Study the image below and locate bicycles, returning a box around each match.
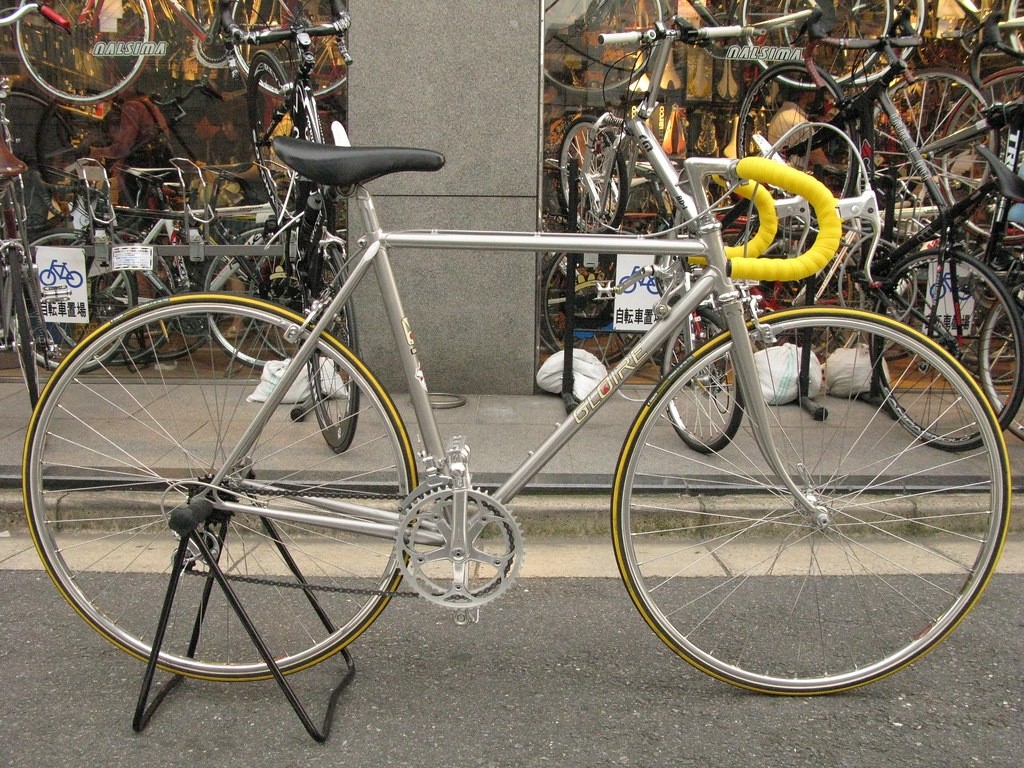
[23,136,1014,745]
[1,1,1024,457]
